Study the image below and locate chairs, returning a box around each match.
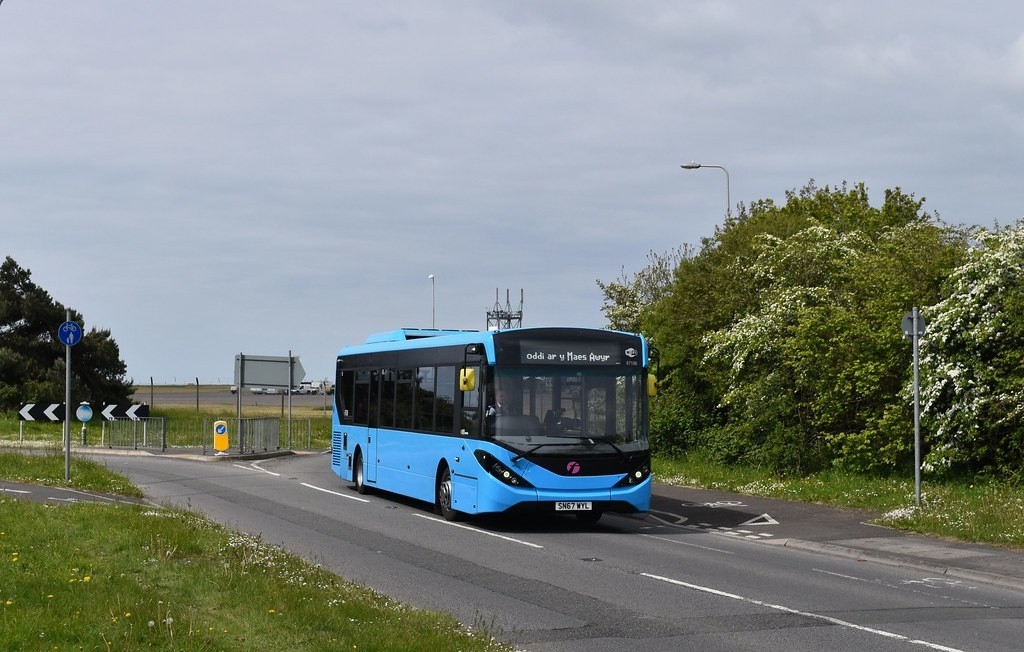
[495,415,545,436]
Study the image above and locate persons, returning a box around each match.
[493,388,521,416]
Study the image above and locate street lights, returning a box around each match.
[680,162,730,220]
[428,274,435,328]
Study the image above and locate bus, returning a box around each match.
[331,326,653,520]
